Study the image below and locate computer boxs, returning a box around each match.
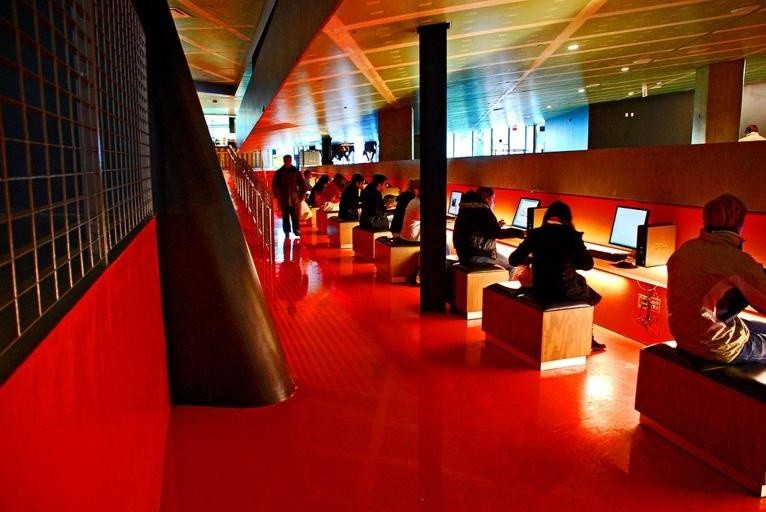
[635,222,677,267]
[527,206,548,235]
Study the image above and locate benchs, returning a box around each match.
[635,339,766,498]
[445,255,593,372]
[308,208,420,284]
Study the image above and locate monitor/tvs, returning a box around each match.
[448,189,463,218]
[608,205,650,249]
[511,197,541,231]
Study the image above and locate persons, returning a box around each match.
[391,179,416,240]
[273,239,310,316]
[307,174,329,205]
[270,155,305,239]
[302,169,314,191]
[666,192,766,368]
[508,200,607,352]
[337,173,364,221]
[358,174,394,230]
[319,173,348,212]
[451,187,528,281]
[399,180,421,244]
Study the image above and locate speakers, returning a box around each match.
[229,117,235,134]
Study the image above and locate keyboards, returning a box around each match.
[587,248,628,262]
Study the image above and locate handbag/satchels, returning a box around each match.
[369,211,388,228]
[509,262,534,288]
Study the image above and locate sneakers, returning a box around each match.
[592,339,606,349]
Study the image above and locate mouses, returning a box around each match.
[617,259,632,267]
